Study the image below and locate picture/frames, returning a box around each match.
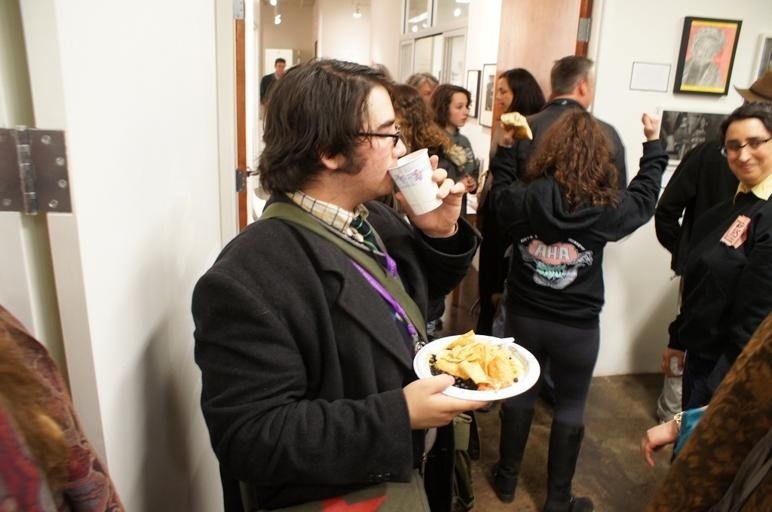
[656,106,731,167]
[675,15,741,97]
[750,33,771,86]
[464,64,496,127]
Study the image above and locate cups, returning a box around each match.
[388,148,443,216]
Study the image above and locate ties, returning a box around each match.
[350,215,384,258]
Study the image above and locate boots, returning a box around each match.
[489,405,536,504]
[543,418,593,512]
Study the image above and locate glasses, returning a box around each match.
[355,126,402,147]
[720,135,772,159]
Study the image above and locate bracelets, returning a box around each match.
[672,410,686,432]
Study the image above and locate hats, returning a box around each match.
[732,69,772,106]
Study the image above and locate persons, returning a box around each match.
[0,306,132,511]
[375,53,670,511]
[650,65,771,424]
[186,52,492,512]
[259,58,289,120]
[659,99,771,471]
[638,313,771,511]
[682,25,727,90]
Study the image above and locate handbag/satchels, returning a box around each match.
[275,469,432,511]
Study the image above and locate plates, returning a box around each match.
[412,335,540,402]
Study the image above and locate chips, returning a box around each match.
[435,329,520,385]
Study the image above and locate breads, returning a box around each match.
[500,109,534,142]
